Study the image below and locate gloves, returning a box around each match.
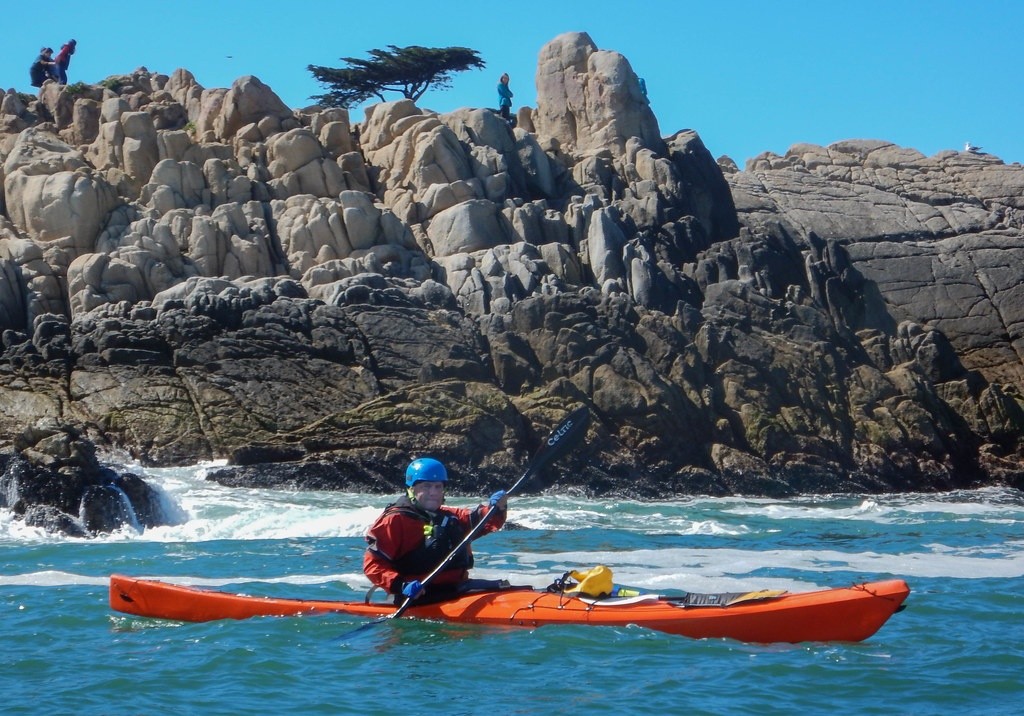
[402,580,426,600]
[488,490,508,512]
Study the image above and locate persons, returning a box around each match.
[363,457,509,607]
[29,39,77,86]
[497,73,514,121]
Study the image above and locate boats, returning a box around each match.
[109,571,909,646]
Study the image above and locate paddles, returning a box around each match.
[348,404,595,639]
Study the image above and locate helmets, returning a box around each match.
[405,458,448,486]
